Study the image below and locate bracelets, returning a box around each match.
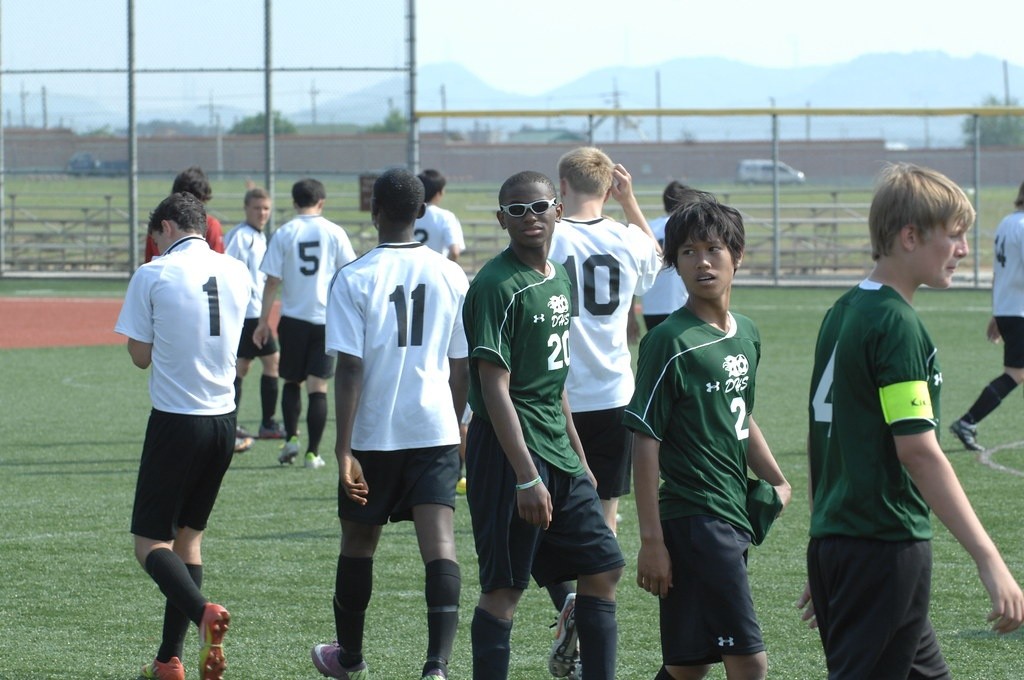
[516,476,542,491]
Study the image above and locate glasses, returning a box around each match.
[501,198,557,218]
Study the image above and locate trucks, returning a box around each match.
[65,152,128,178]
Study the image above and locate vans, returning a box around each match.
[738,159,805,188]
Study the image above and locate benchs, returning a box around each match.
[0,187,996,275]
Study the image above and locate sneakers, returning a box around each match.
[197,602,232,680]
[278,436,300,464]
[311,641,367,680]
[949,420,985,451]
[258,420,300,438]
[419,667,446,680]
[548,592,579,677]
[234,436,254,452]
[141,655,185,680]
[304,452,326,467]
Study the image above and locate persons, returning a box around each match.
[311,167,470,680]
[548,147,663,679]
[417,169,473,495]
[462,171,627,680]
[795,162,1024,680]
[641,181,689,331]
[223,188,301,452]
[112,194,253,680]
[948,182,1024,451]
[252,178,357,469]
[620,189,792,680]
[145,166,255,452]
